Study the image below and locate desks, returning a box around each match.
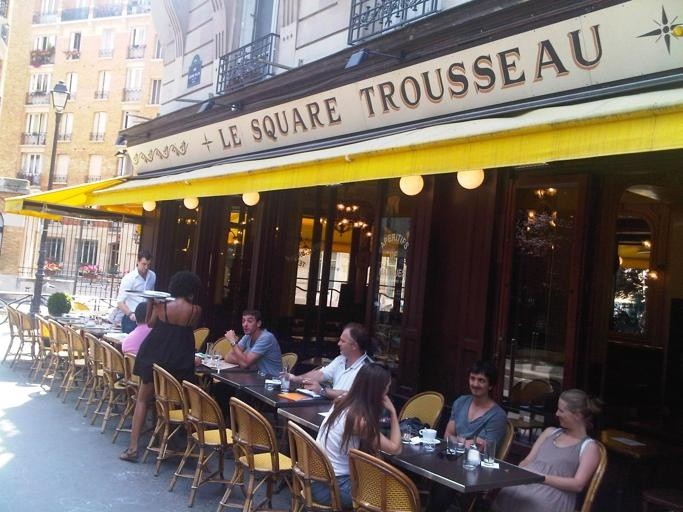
[209,370,277,391]
[278,403,330,432]
[245,385,333,407]
[398,448,548,493]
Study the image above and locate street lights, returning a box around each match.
[16,81,70,322]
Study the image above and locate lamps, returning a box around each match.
[184,198,199,210]
[143,201,156,211]
[320,185,372,237]
[457,169,485,190]
[242,192,260,206]
[399,175,424,196]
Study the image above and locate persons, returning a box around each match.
[423,360,508,512]
[491,386,606,512]
[117,250,157,334]
[224,308,284,377]
[118,269,204,462]
[278,321,376,400]
[121,301,153,356]
[309,362,405,509]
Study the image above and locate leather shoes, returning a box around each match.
[119,449,139,461]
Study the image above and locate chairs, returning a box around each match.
[142,364,211,477]
[281,353,299,372]
[397,391,444,430]
[4,306,208,442]
[169,381,238,508]
[463,418,515,507]
[287,420,344,511]
[217,398,295,511]
[575,441,608,509]
[211,336,238,359]
[348,448,422,511]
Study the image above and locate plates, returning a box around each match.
[60,310,122,331]
[141,290,171,298]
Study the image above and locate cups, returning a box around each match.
[481,438,497,465]
[461,443,481,470]
[397,423,466,457]
[193,340,227,376]
[255,363,293,395]
[467,472,481,487]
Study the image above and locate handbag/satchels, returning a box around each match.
[400,417,429,437]
